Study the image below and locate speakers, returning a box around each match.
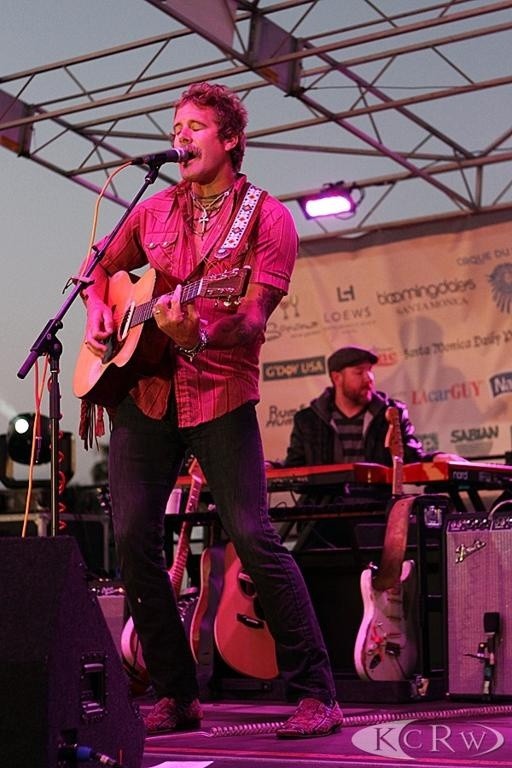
[1,536,145,767]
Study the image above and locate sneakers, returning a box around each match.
[274,696,344,739]
[143,694,205,734]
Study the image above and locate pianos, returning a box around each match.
[385,454,511,493]
[174,460,387,495]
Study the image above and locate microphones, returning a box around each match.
[132,147,196,166]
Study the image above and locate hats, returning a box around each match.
[327,346,380,374]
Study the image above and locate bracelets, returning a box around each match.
[176,335,207,357]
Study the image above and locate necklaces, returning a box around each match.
[190,185,232,235]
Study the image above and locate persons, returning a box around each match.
[266,347,425,552]
[79,81,344,739]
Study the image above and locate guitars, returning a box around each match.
[184,505,204,666]
[199,542,283,688]
[70,267,250,409]
[119,456,209,681]
[354,406,420,681]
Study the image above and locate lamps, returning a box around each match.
[300,187,355,220]
